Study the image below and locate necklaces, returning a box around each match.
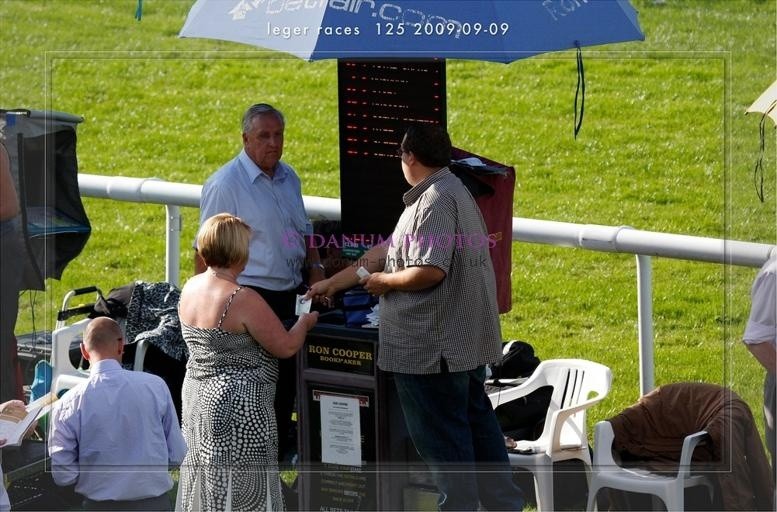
[207,269,235,279]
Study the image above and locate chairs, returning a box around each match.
[587,382,740,511]
[49,283,178,395]
[14,285,102,366]
[488,358,613,511]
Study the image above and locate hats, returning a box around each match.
[401,124,452,169]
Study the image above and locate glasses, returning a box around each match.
[397,148,405,155]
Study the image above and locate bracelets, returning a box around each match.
[307,260,326,272]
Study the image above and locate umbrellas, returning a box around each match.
[177,0,645,64]
[744,80,776,129]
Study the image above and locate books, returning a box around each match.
[0,399,44,448]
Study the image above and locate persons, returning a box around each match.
[192,102,328,470]
[0,141,28,403]
[0,438,12,512]
[46,316,189,512]
[303,120,526,511]
[174,212,320,512]
[741,255,776,475]
